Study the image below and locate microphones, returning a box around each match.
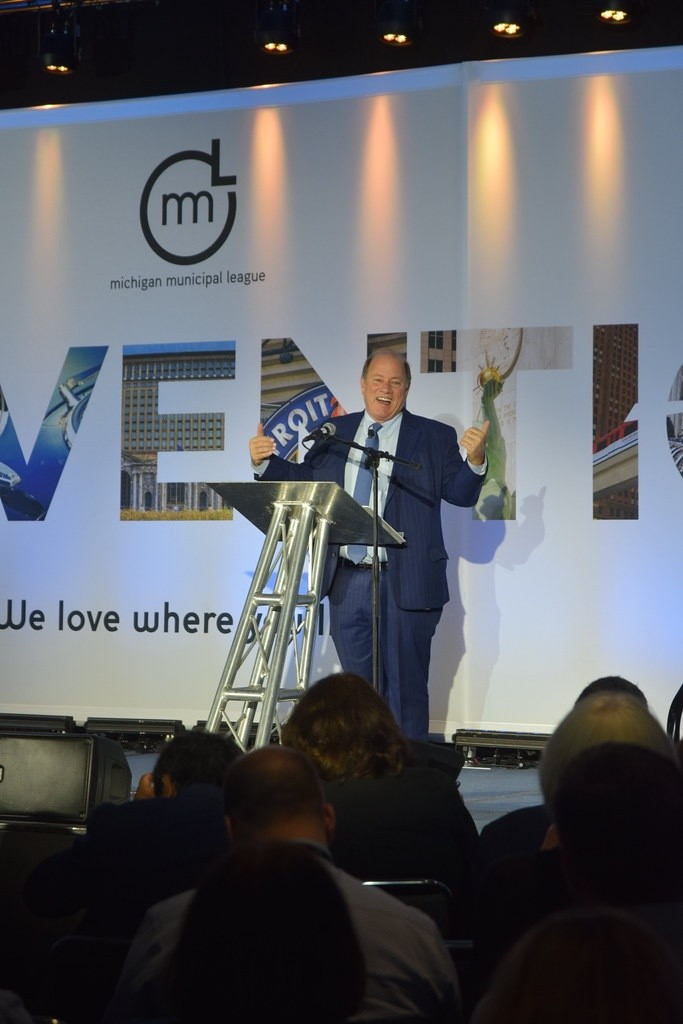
[303,423,337,443]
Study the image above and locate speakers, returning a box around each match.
[0,731,134,828]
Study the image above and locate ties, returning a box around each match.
[346,423,382,565]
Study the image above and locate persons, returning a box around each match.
[467,690,683,935]
[99,745,464,1024]
[284,674,479,893]
[22,732,245,938]
[473,907,683,1024]
[554,744,683,956]
[168,841,368,1024]
[249,350,491,740]
[469,677,683,884]
